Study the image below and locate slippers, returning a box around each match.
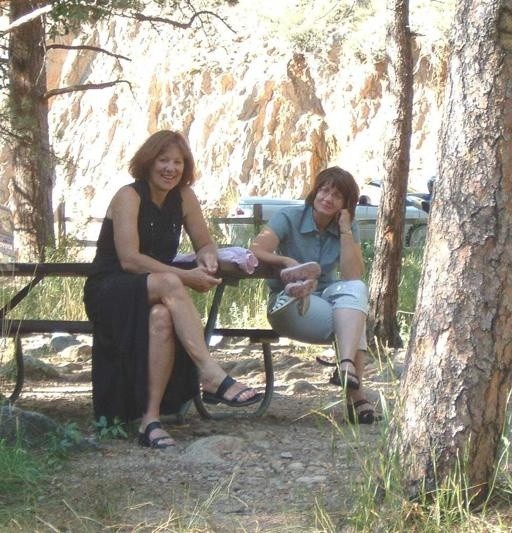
[203,376,263,407]
[139,422,176,448]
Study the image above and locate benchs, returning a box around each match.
[0,315,204,407]
[210,325,279,419]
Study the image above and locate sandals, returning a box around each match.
[268,261,321,316]
[348,399,383,422]
[330,359,360,388]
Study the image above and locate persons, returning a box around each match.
[251,166,382,424]
[83,129,265,449]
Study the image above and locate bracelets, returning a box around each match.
[340,230,352,235]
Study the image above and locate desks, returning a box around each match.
[0,254,286,421]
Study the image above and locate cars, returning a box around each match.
[178,175,435,249]
